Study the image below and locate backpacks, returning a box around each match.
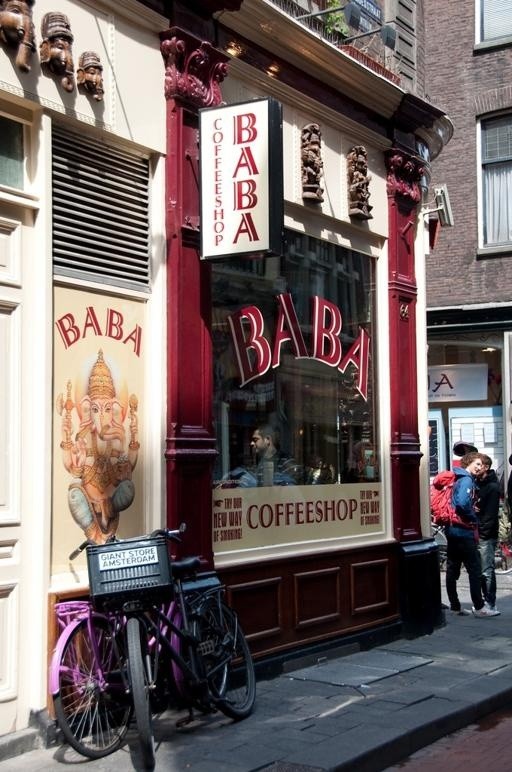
[429,470,475,529]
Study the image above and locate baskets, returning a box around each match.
[86,536,172,606]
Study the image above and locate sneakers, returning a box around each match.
[451,608,472,616]
[472,601,501,617]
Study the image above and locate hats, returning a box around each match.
[454,441,478,456]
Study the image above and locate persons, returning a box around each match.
[470,454,501,611]
[445,452,502,618]
[239,425,302,485]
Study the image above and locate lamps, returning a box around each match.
[293,1,398,53]
